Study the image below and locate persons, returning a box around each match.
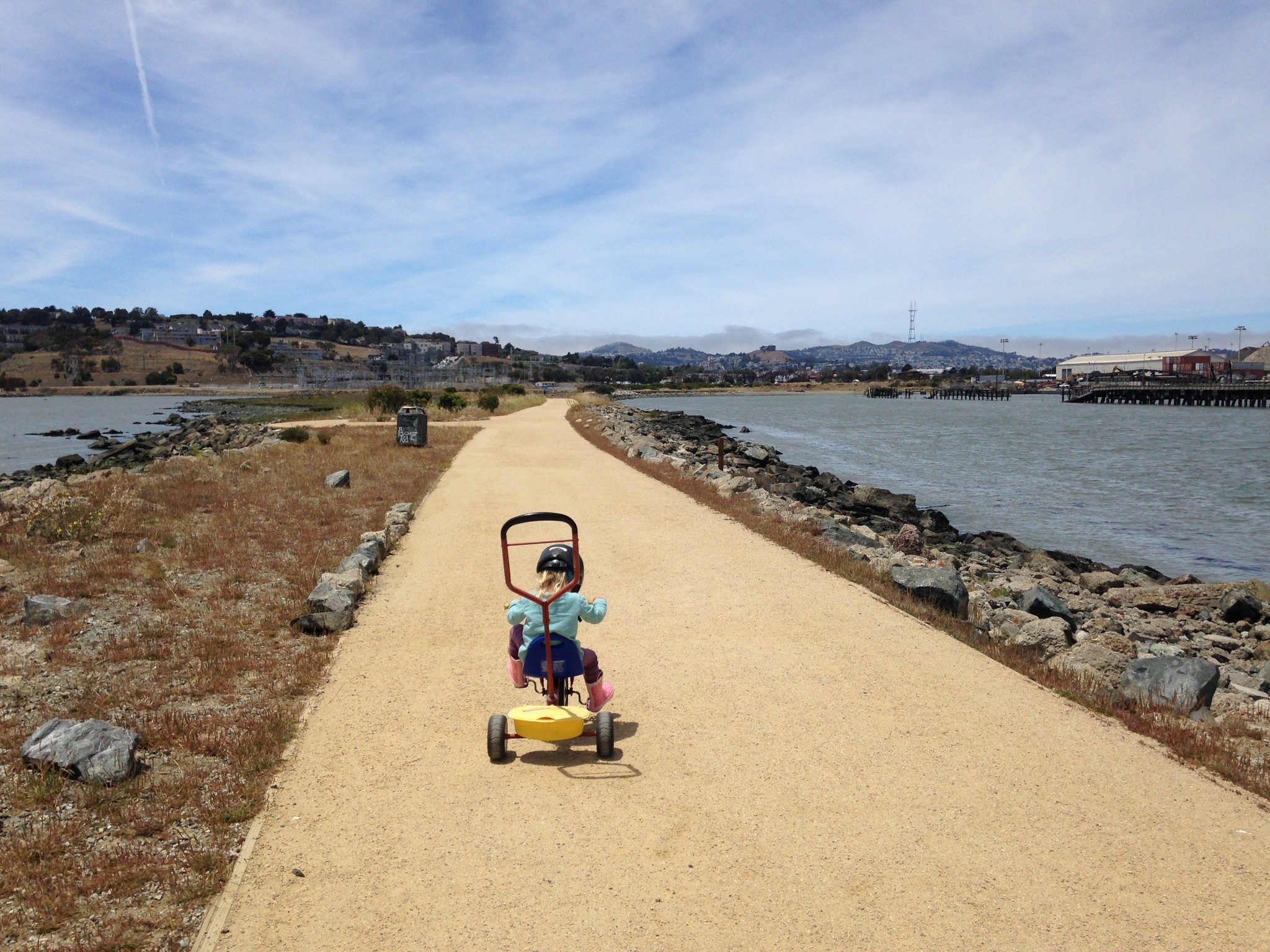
[506,544,614,713]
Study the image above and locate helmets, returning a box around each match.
[536,544,584,593]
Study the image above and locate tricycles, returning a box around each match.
[486,511,616,760]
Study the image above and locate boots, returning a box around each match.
[507,652,527,688]
[585,669,614,713]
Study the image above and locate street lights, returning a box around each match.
[1188,335,1198,350]
[1039,342,1042,377]
[1234,325,1247,362]
[1000,338,1009,374]
